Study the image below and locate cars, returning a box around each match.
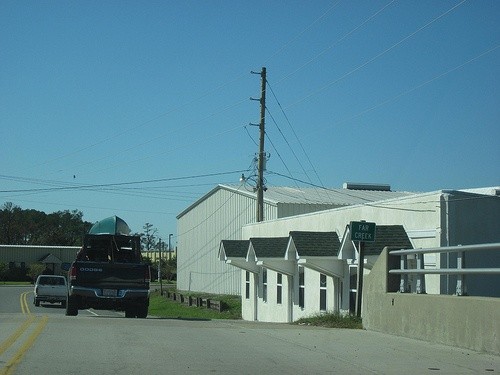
[33,275,67,307]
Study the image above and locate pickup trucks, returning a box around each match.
[65,234,152,320]
